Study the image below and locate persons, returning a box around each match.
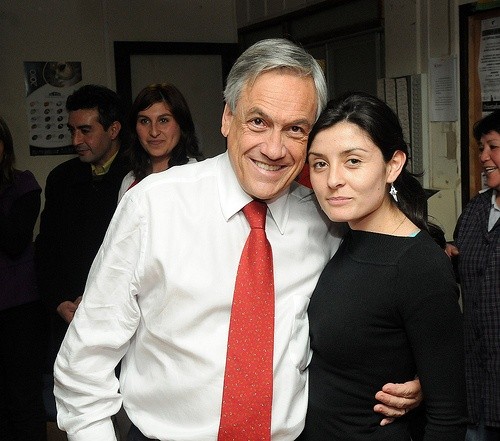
[116,82,204,206]
[445,108,500,441]
[53,38,421,441]
[306,91,469,441]
[0,116,47,441]
[30,84,136,396]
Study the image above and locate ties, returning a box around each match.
[218,201,275,441]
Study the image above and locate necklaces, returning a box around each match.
[391,216,407,233]
